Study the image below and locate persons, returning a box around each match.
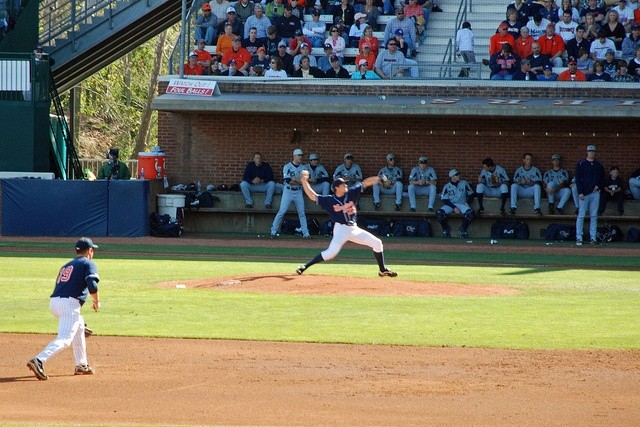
[333,153,363,187]
[268,148,312,240]
[475,158,511,217]
[178,0,444,78]
[575,144,601,247]
[296,170,398,277]
[543,153,572,215]
[96,148,131,180]
[482,0,640,82]
[436,168,475,239]
[407,157,438,212]
[629,175,640,200]
[597,163,624,216]
[569,175,579,216]
[510,153,542,217]
[372,153,405,211]
[240,151,276,209]
[304,153,330,196]
[27,236,100,381]
[455,21,476,77]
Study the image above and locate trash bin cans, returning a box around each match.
[157,194,185,237]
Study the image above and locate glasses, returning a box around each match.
[287,8,292,11]
[331,30,338,32]
[503,29,507,31]
[419,160,428,164]
[397,13,403,15]
[363,64,368,66]
[595,65,602,66]
[390,158,395,161]
[390,44,396,45]
[314,13,320,16]
[270,62,276,64]
[205,10,211,12]
[527,63,531,66]
[225,21,231,25]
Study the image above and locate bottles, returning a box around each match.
[84,168,89,180]
[229,64,234,75]
[197,179,202,191]
[140,168,145,178]
[525,71,530,80]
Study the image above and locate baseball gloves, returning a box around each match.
[487,173,502,187]
[79,322,95,342]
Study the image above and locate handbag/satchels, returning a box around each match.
[391,219,431,237]
[321,219,335,236]
[283,218,320,235]
[588,224,623,241]
[490,220,530,240]
[357,217,391,236]
[184,191,214,207]
[545,223,584,242]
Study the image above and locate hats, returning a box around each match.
[586,145,596,151]
[227,7,236,13]
[354,12,366,20]
[362,42,371,48]
[499,23,508,28]
[189,51,198,57]
[418,157,428,161]
[448,169,460,177]
[547,24,555,27]
[333,178,349,186]
[110,149,118,154]
[386,153,394,158]
[607,49,615,54]
[278,42,286,47]
[395,29,403,35]
[301,42,308,48]
[631,24,640,29]
[344,154,353,159]
[75,236,99,251]
[293,149,303,154]
[502,43,512,49]
[258,47,265,51]
[335,16,343,23]
[324,43,332,49]
[228,59,236,64]
[232,36,241,41]
[310,153,318,159]
[552,154,560,159]
[331,55,338,62]
[312,9,320,14]
[543,65,552,69]
[199,39,206,44]
[284,3,292,8]
[203,3,211,10]
[569,60,577,64]
[359,59,366,65]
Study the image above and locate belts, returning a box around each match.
[287,187,301,191]
[488,186,500,188]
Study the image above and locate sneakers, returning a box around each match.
[459,227,468,239]
[245,202,254,208]
[534,208,542,216]
[74,364,94,375]
[590,240,599,246]
[395,204,402,212]
[576,240,582,246]
[510,208,517,215]
[373,202,382,211]
[296,265,305,275]
[270,230,277,238]
[442,225,451,238]
[378,268,398,277]
[302,232,311,238]
[266,203,272,209]
[27,357,48,381]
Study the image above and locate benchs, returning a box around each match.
[185,186,639,238]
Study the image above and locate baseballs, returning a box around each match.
[302,170,309,176]
